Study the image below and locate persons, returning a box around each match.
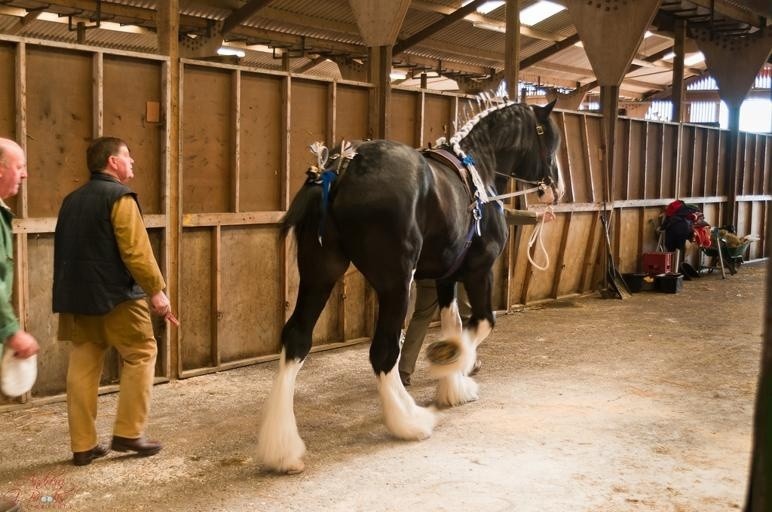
[50,134,172,467]
[0,136,43,358]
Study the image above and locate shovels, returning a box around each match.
[600,217,633,299]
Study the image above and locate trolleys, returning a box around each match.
[703,242,750,274]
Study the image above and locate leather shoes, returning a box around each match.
[73,444,111,465]
[111,436,163,453]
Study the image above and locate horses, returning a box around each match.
[255,96,568,476]
